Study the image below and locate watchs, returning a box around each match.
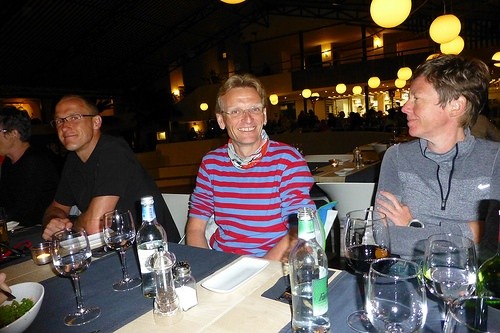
[408,219,424,229]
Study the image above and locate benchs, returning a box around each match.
[160,183,375,259]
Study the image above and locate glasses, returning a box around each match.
[221,106,263,118]
[50,113,96,130]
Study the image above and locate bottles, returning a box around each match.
[145,246,180,317]
[474,210,500,333]
[173,261,198,312]
[289,207,331,333]
[135,196,169,298]
[353,146,362,170]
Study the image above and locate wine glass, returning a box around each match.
[102,209,142,292]
[50,227,101,326]
[422,233,476,323]
[343,210,391,333]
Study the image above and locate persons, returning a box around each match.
[373,54,500,263]
[187,106,407,141]
[186,74,317,264]
[42,94,181,244]
[470,113,500,142]
[0,105,64,227]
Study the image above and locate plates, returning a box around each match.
[201,256,270,293]
[334,167,355,176]
[59,231,103,248]
[0,221,19,233]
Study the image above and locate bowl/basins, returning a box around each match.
[0,282,44,333]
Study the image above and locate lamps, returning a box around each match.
[370,0,412,28]
[429,0,465,55]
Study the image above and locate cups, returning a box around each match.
[0,219,9,245]
[366,258,428,333]
[30,241,51,265]
[292,208,327,299]
[442,296,500,333]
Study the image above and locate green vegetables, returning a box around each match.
[0,297,33,331]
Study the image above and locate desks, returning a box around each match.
[312,158,380,187]
[0,227,445,333]
